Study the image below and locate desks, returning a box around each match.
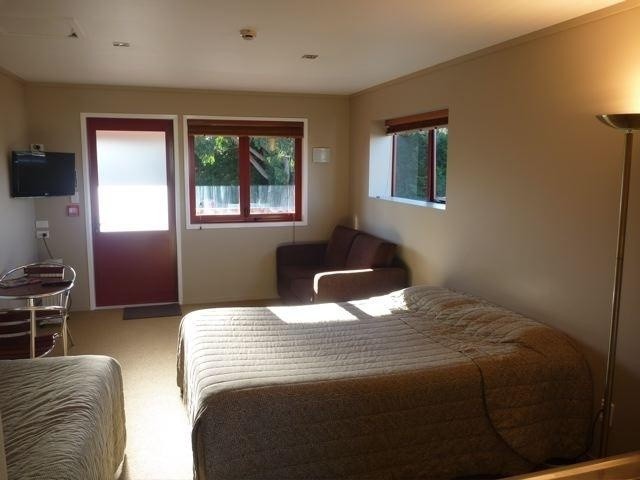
[0,276,74,360]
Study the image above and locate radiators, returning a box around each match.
[39,256,64,317]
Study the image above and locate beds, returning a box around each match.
[172,283,603,479]
[1,355,129,479]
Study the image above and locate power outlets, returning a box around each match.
[37,230,50,239]
[597,397,616,427]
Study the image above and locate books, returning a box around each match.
[0,276,72,290]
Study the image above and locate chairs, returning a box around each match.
[0,260,77,347]
[0,304,67,360]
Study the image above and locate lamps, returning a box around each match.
[585,107,640,462]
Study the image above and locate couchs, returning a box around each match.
[274,225,408,306]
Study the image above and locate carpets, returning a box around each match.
[121,301,183,320]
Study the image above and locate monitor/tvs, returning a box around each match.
[8,150,75,198]
